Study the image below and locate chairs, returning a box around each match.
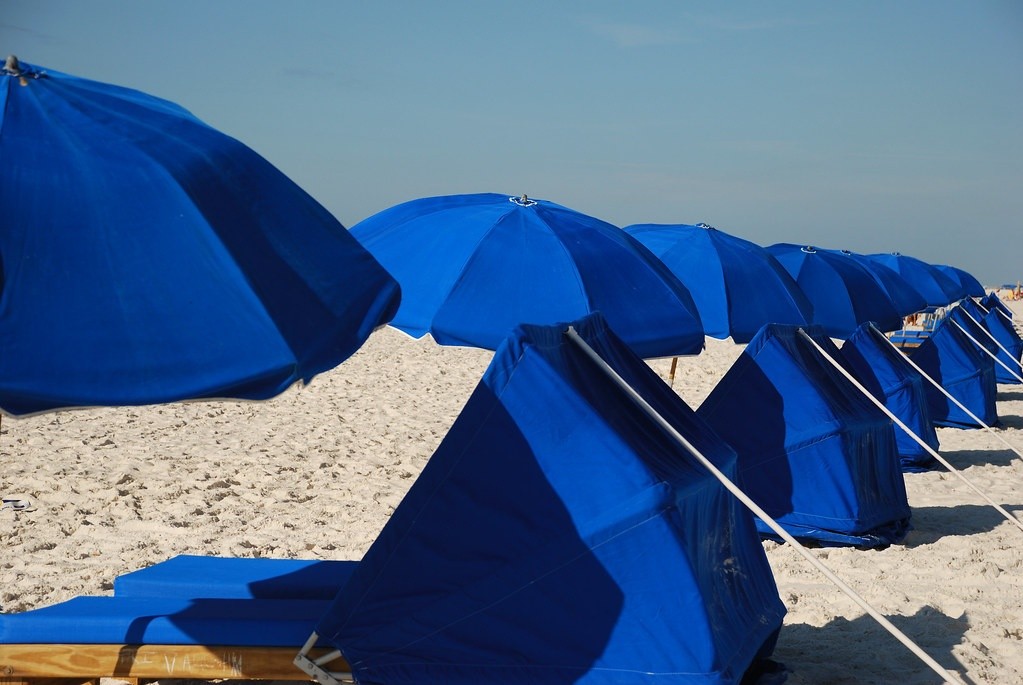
[0,552,357,684]
[888,328,930,352]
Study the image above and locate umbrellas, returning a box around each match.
[762,243,986,341]
[617,222,814,345]
[0,56,402,421]
[345,193,706,360]
